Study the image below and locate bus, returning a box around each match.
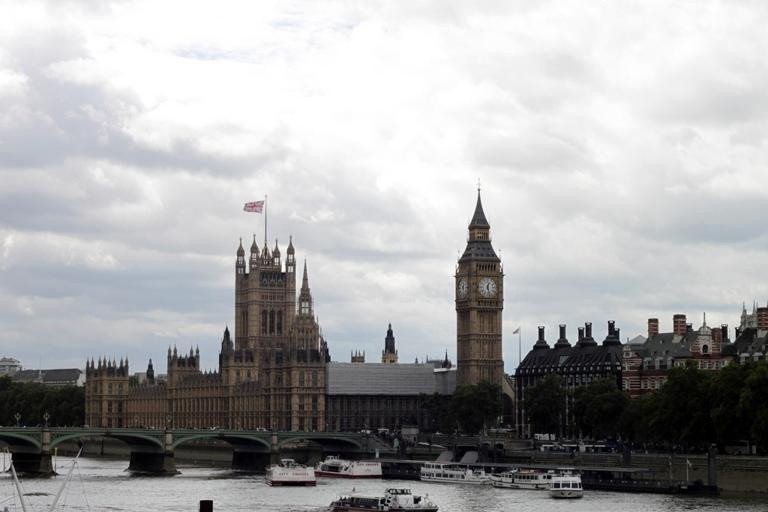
[711,438,751,456]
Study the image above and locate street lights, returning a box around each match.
[14,412,22,427]
[43,410,50,427]
[432,417,436,433]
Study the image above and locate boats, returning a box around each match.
[315,455,383,479]
[486,467,551,490]
[265,458,317,488]
[363,457,424,480]
[672,459,722,495]
[575,464,672,491]
[546,470,585,498]
[327,485,440,512]
[420,461,491,485]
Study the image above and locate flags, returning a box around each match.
[512,328,520,335]
[243,199,265,214]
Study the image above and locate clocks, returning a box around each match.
[458,278,497,298]
